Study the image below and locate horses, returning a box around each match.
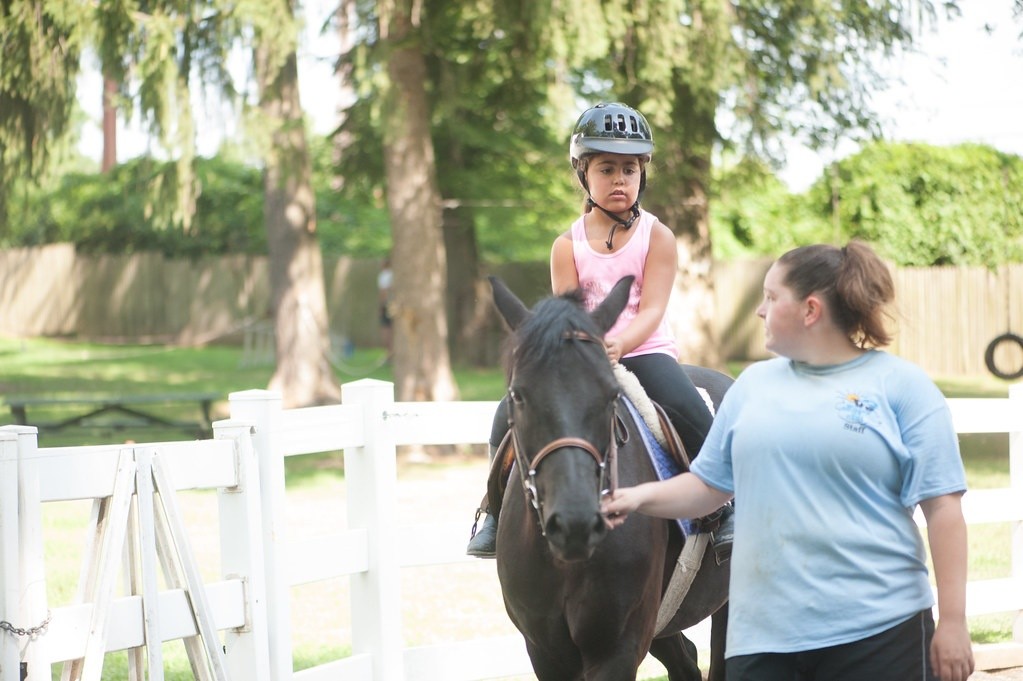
[486,275,735,681]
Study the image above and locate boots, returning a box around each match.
[467,444,500,557]
[714,505,734,554]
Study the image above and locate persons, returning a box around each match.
[598,241,974,681]
[466,102,735,566]
[375,256,396,364]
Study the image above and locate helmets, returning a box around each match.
[569,101,654,172]
